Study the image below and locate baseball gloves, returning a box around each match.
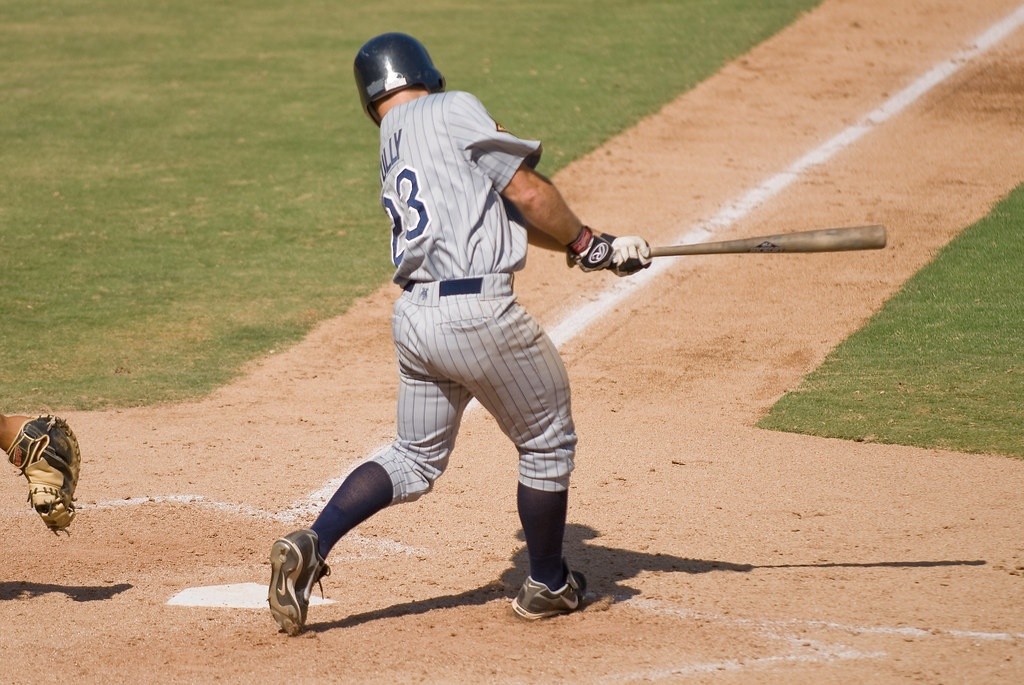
[6,415,80,537]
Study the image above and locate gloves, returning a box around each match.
[565,226,615,271]
[600,233,651,277]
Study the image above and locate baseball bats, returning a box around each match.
[565,223,887,265]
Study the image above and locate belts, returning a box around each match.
[403,278,482,296]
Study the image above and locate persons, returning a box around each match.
[266,32,652,638]
[0,414,46,470]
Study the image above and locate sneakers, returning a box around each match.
[269,529,331,636]
[512,557,587,620]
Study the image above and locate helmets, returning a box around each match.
[354,32,445,127]
